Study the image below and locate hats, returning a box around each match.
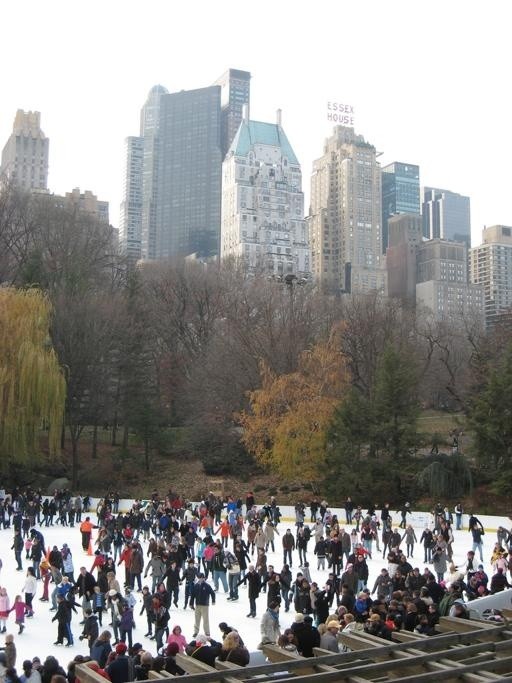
[295,613,304,621]
[359,591,366,597]
[367,613,380,622]
[116,643,126,654]
[328,621,342,629]
[168,642,178,652]
[269,602,278,608]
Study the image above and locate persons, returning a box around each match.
[1,482,512,682]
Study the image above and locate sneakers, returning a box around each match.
[65,643,72,646]
[39,597,49,601]
[54,641,63,644]
[174,602,195,610]
[150,636,155,640]
[1,627,6,633]
[19,625,24,634]
[193,633,197,637]
[227,597,237,600]
